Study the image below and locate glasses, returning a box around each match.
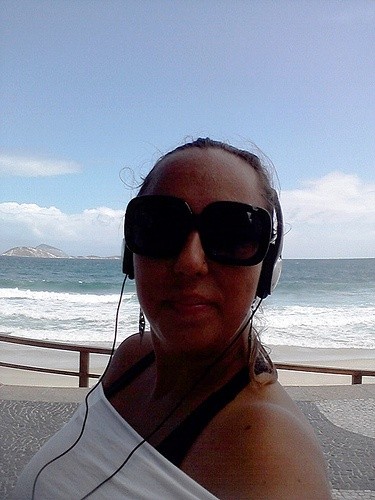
[124,194,273,267]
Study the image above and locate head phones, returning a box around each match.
[122,186,284,298]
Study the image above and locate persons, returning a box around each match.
[14,138,332,500]
